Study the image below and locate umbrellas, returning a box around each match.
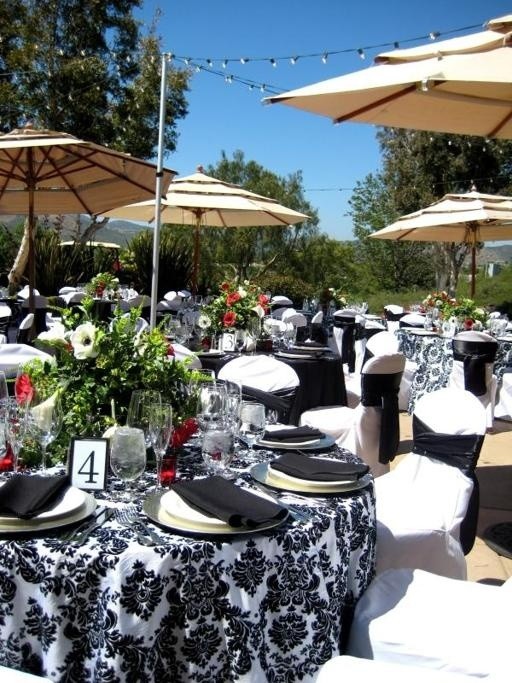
[365,183,512,298]
[258,12,512,140]
[0,121,180,336]
[93,164,313,299]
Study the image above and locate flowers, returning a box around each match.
[209,279,272,331]
[91,270,119,291]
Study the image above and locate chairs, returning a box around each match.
[349,565,512,683]
[315,656,512,683]
[1,284,512,426]
[296,351,408,479]
[369,387,490,580]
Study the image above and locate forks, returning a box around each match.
[114,506,163,546]
[44,507,110,545]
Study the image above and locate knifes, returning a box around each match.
[244,480,308,524]
[72,507,117,549]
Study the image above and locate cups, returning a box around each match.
[189,369,278,473]
[164,319,188,344]
[125,389,162,449]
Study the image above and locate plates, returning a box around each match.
[249,457,371,494]
[253,432,335,451]
[276,351,312,360]
[140,482,290,537]
[1,485,98,532]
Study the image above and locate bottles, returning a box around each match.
[209,333,222,350]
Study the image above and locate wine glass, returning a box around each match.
[185,311,204,345]
[109,403,173,502]
[4,385,64,476]
[283,330,297,350]
